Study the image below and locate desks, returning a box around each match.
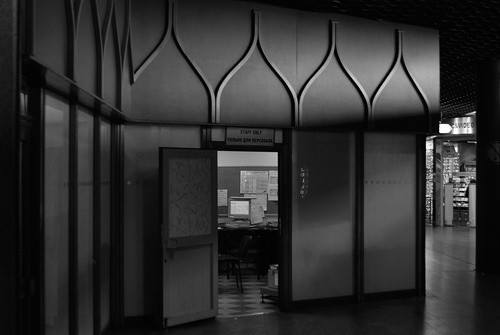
[217,223,280,280]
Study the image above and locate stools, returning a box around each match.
[218,253,243,292]
